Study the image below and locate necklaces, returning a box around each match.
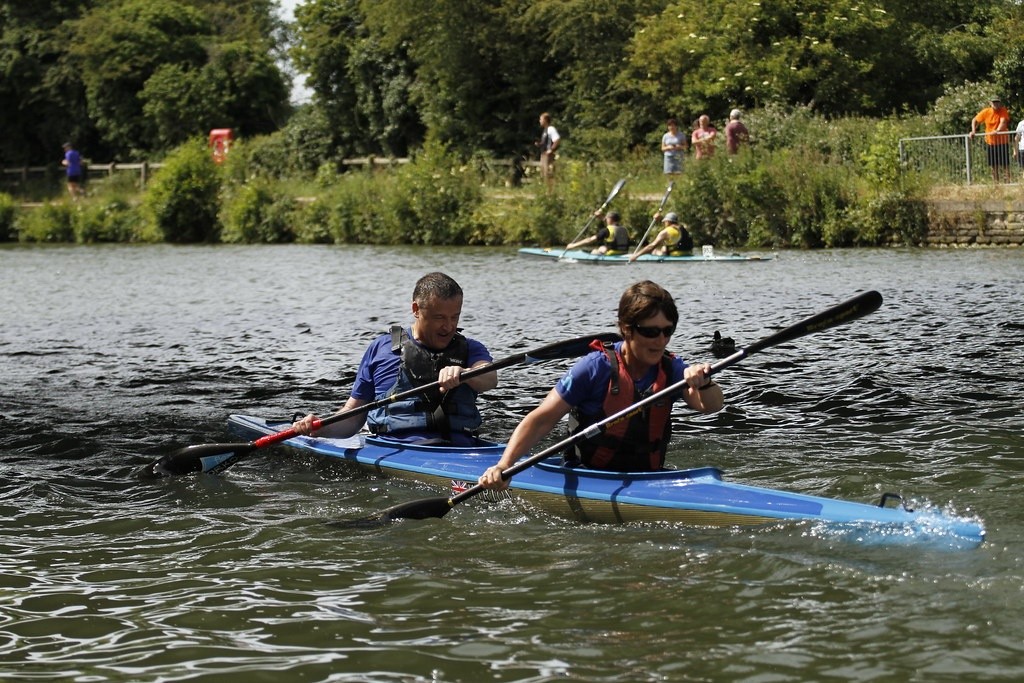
[635,383,650,418]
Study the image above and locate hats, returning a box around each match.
[989,95,1001,102]
[661,212,678,222]
[603,211,621,221]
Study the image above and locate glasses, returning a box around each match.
[635,325,676,337]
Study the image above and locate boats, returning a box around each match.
[222,413,986,554]
[516,247,781,264]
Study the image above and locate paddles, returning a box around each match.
[373,289,883,518]
[155,331,620,475]
[551,176,628,264]
[627,180,675,266]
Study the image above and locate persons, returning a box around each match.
[535,110,562,197]
[630,211,692,260]
[1013,116,1024,171]
[969,97,1011,184]
[62,139,85,204]
[726,109,749,154]
[660,118,688,191]
[566,209,629,255]
[477,282,723,491]
[691,114,717,161]
[292,272,498,439]
